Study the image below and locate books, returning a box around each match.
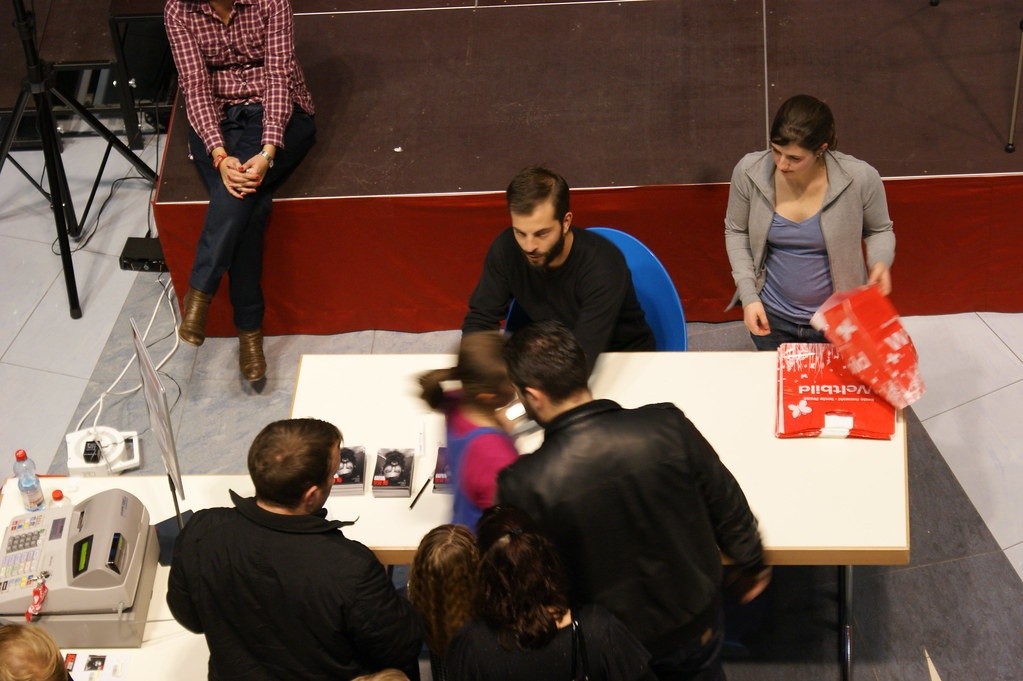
[432,446,455,495]
[330,445,367,496]
[371,447,414,498]
[496,394,537,438]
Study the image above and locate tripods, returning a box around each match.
[0,0,158,319]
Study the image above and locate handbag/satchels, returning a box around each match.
[811,284,925,410]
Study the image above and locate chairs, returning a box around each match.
[504,228,688,352]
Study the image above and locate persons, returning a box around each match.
[163,0,318,384]
[461,165,658,386]
[497,313,767,681]
[348,518,659,681]
[335,448,356,481]
[381,450,405,483]
[168,419,426,681]
[422,330,525,531]
[725,94,896,351]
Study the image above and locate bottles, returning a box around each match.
[14,450,46,513]
[49,490,73,508]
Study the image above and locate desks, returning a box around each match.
[1,475,256,680]
[287,352,910,680]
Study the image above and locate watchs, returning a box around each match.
[259,151,274,168]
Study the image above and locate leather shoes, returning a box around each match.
[177,287,212,346]
[238,329,267,382]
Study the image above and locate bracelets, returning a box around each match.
[213,153,227,168]
